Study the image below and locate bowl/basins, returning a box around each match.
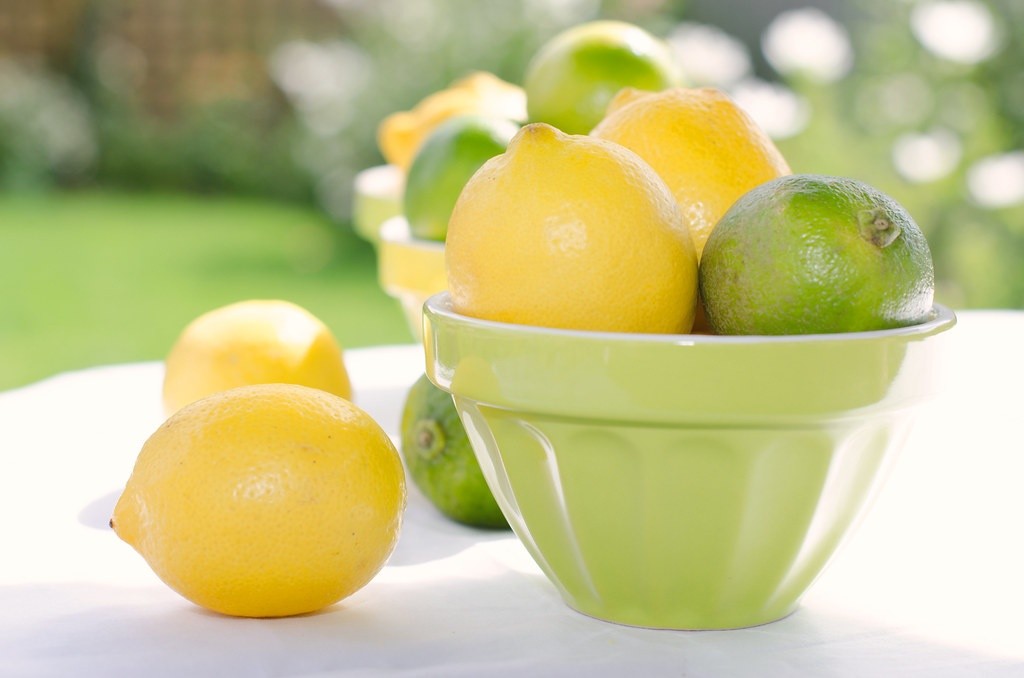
[421,289,956,631]
[375,214,445,339]
